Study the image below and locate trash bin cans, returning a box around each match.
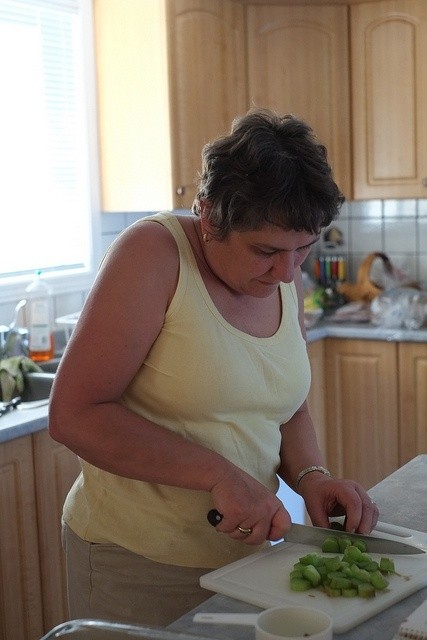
[293,464,331,493]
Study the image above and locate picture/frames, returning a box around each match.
[127,449,425,640]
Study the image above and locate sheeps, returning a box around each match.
[0,325,29,358]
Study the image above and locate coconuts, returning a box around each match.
[289,523,396,600]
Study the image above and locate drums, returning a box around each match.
[207,509,426,554]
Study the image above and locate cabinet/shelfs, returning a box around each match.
[25,266,55,361]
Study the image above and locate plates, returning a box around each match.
[248,1,353,211]
[305,329,324,473]
[347,0,426,203]
[0,417,47,639]
[398,332,427,469]
[325,329,399,490]
[33,416,91,633]
[95,0,244,212]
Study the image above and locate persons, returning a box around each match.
[48,106,379,629]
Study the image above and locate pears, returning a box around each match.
[24,269,55,362]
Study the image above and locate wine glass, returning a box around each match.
[236,526,251,537]
[370,500,376,504]
